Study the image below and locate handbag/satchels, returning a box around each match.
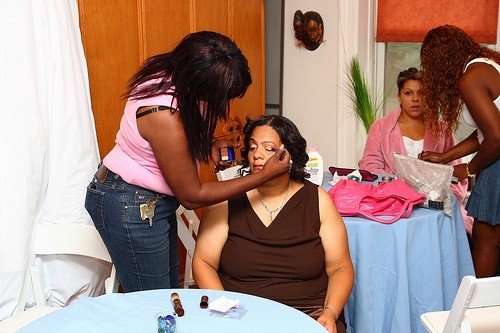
[325,178,426,225]
[393,151,454,218]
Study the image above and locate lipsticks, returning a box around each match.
[170,292,185,317]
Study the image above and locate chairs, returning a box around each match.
[0,217,116,333]
[420,274,500,333]
[175,203,200,288]
[216,164,250,181]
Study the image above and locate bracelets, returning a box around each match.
[466,164,476,178]
[323,306,339,320]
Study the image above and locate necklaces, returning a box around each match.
[257,179,291,222]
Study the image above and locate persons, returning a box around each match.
[192,114,355,333]
[416,23,500,278]
[84,29,295,295]
[356,67,474,240]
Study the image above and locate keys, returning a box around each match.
[141,204,155,226]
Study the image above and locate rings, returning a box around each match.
[290,160,293,164]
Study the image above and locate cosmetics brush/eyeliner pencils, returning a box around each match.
[270,146,286,153]
[419,150,424,160]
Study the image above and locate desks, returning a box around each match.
[16,287,330,333]
[319,171,477,332]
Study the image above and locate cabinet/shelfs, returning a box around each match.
[78,0,266,292]
